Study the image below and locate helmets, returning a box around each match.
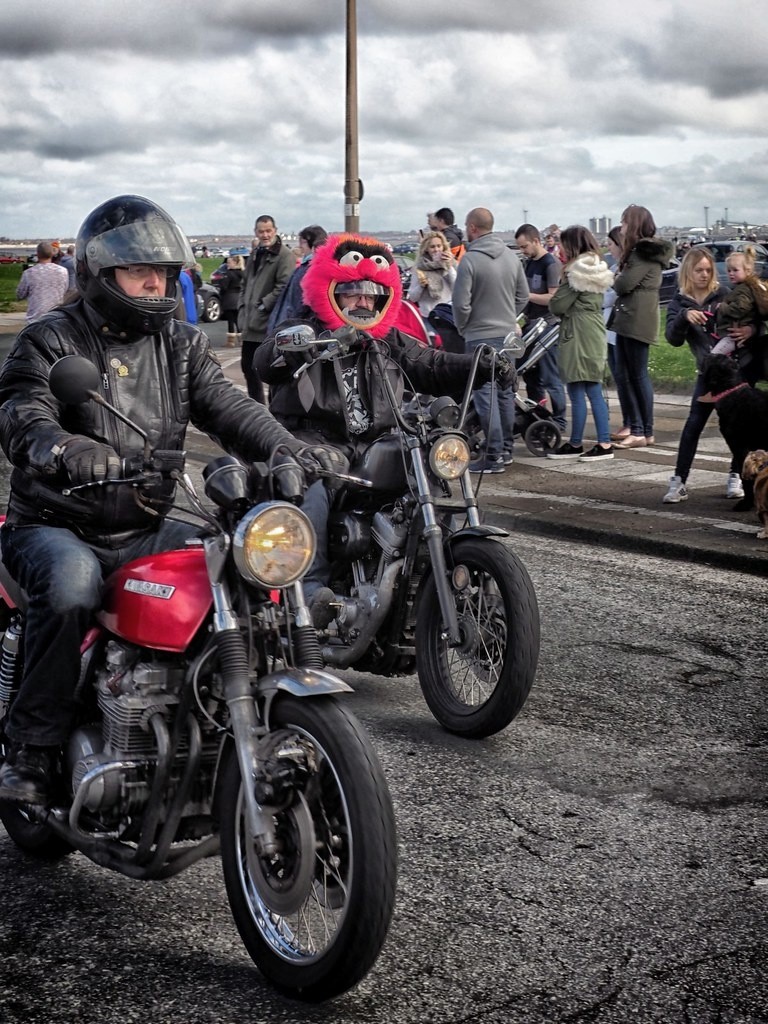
[75,196,196,333]
[334,281,389,319]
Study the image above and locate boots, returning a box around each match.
[237,333,243,347]
[222,332,236,348]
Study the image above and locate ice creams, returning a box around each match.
[415,269,425,282]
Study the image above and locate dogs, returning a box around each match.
[742,449,768,540]
[698,352,768,515]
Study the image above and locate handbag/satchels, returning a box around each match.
[430,303,454,325]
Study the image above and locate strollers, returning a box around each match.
[427,299,564,457]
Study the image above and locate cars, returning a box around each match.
[0,251,26,264]
[392,241,420,292]
[192,241,249,324]
[602,233,768,305]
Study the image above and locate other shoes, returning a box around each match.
[698,392,715,403]
[614,437,646,448]
[646,436,655,444]
[609,433,629,439]
[307,587,336,644]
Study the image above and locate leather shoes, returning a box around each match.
[0,740,59,805]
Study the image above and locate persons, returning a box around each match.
[237,215,296,404]
[662,245,768,502]
[740,234,756,242]
[0,196,351,806]
[547,224,614,461]
[407,207,677,448]
[16,241,79,329]
[252,231,519,629]
[254,227,328,401]
[451,208,529,474]
[175,245,245,348]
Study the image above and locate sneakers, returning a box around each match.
[662,476,689,502]
[547,443,584,459]
[579,444,614,461]
[502,451,513,466]
[468,457,506,473]
[727,473,745,498]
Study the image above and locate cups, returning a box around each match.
[432,251,441,262]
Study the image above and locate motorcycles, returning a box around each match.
[0,353,403,1004]
[206,323,540,741]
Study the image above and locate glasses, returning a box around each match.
[341,291,379,304]
[112,265,178,277]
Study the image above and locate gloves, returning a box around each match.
[294,445,350,490]
[284,343,330,371]
[482,352,519,393]
[60,438,121,486]
